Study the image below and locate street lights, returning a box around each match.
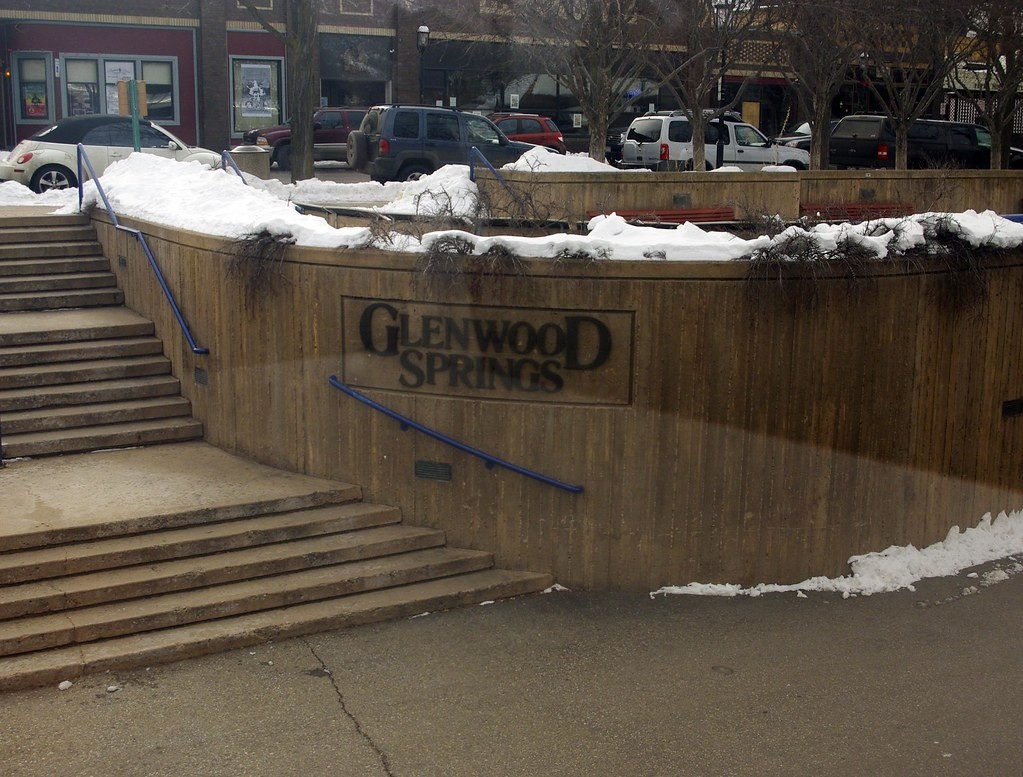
[416,25,430,104]
[860,52,870,115]
[713,0,736,169]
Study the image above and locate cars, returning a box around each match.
[765,120,839,150]
[607,112,644,166]
[0,114,228,194]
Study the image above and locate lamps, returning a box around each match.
[417,26,430,57]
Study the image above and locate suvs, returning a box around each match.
[243,107,368,170]
[347,103,559,186]
[621,109,810,171]
[471,113,566,155]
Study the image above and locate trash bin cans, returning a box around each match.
[227,144,272,180]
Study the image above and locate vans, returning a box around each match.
[828,114,1023,170]
